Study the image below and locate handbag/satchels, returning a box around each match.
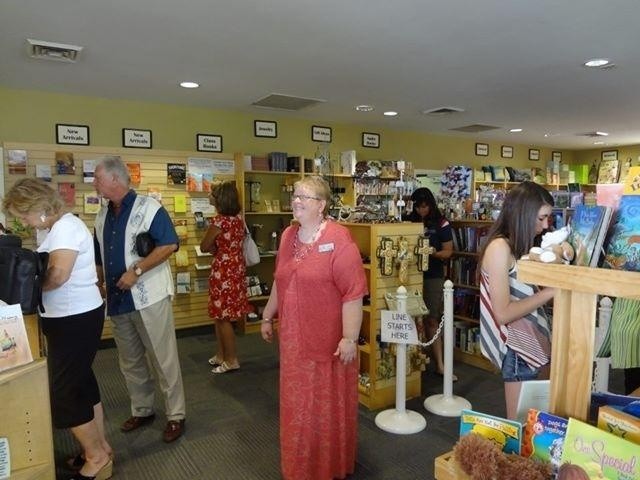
[242,235,260,268]
[1,236,50,316]
[138,233,181,256]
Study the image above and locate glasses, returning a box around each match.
[291,195,321,202]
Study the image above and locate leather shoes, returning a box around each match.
[163,418,185,443]
[119,412,154,431]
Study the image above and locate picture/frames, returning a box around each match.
[123,128,152,149]
[529,149,540,161]
[56,124,89,145]
[601,150,618,162]
[501,145,513,158]
[552,151,562,162]
[476,143,488,156]
[197,134,222,152]
[362,132,380,148]
[312,125,332,143]
[254,120,277,138]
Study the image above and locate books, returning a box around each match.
[443,225,491,356]
[459,391,640,480]
[553,166,640,272]
[252,151,300,172]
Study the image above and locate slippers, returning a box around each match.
[207,356,240,374]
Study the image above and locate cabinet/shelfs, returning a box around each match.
[449,219,500,376]
[472,166,559,203]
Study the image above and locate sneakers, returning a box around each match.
[436,368,458,383]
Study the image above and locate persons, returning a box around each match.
[199,181,252,375]
[259,174,370,480]
[91,156,187,443]
[402,187,458,383]
[0,176,113,480]
[475,180,556,421]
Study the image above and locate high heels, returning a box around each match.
[69,450,115,480]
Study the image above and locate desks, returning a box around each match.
[0,311,60,480]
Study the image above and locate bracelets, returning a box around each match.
[344,338,359,344]
[262,318,273,324]
